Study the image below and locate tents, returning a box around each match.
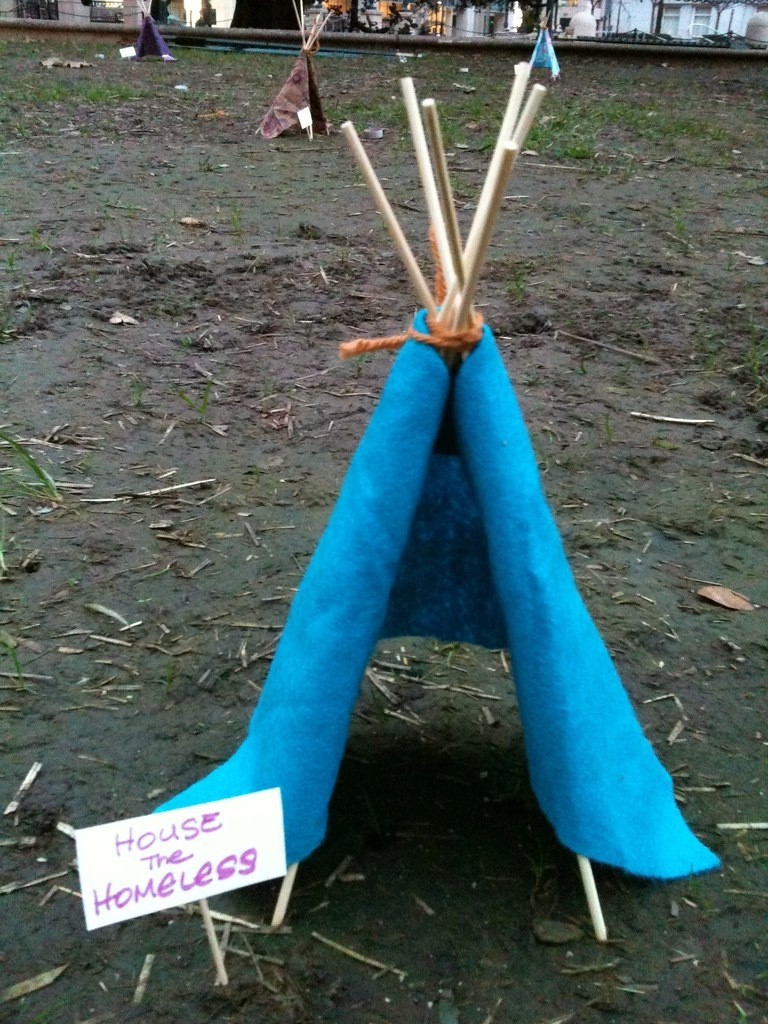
[253,49,331,139]
[528,28,561,81]
[133,16,179,63]
[147,298,725,940]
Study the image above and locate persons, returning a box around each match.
[150,0,171,24]
[200,0,212,27]
